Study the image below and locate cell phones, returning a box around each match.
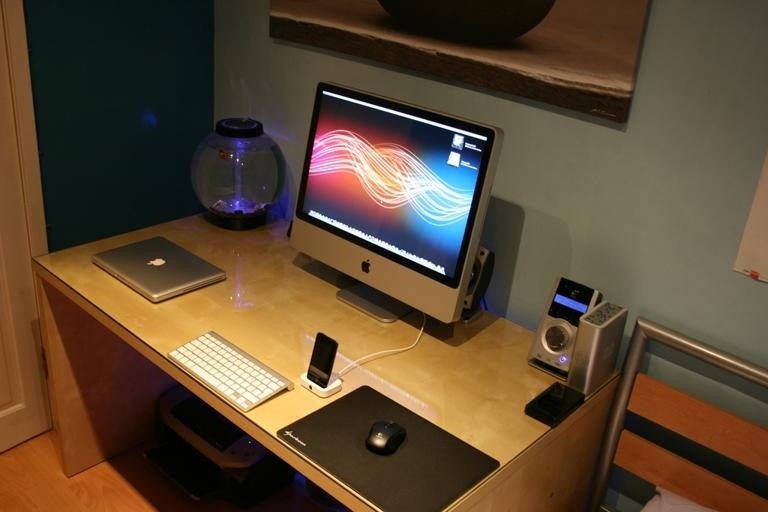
[307,332,338,388]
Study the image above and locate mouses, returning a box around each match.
[365,420,407,455]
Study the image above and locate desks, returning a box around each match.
[32,205,623,511]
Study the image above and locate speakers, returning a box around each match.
[461,245,495,323]
[527,276,603,382]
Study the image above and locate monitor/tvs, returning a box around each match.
[289,79,504,323]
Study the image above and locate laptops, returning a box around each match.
[92,237,226,303]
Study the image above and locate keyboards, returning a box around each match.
[167,330,294,413]
